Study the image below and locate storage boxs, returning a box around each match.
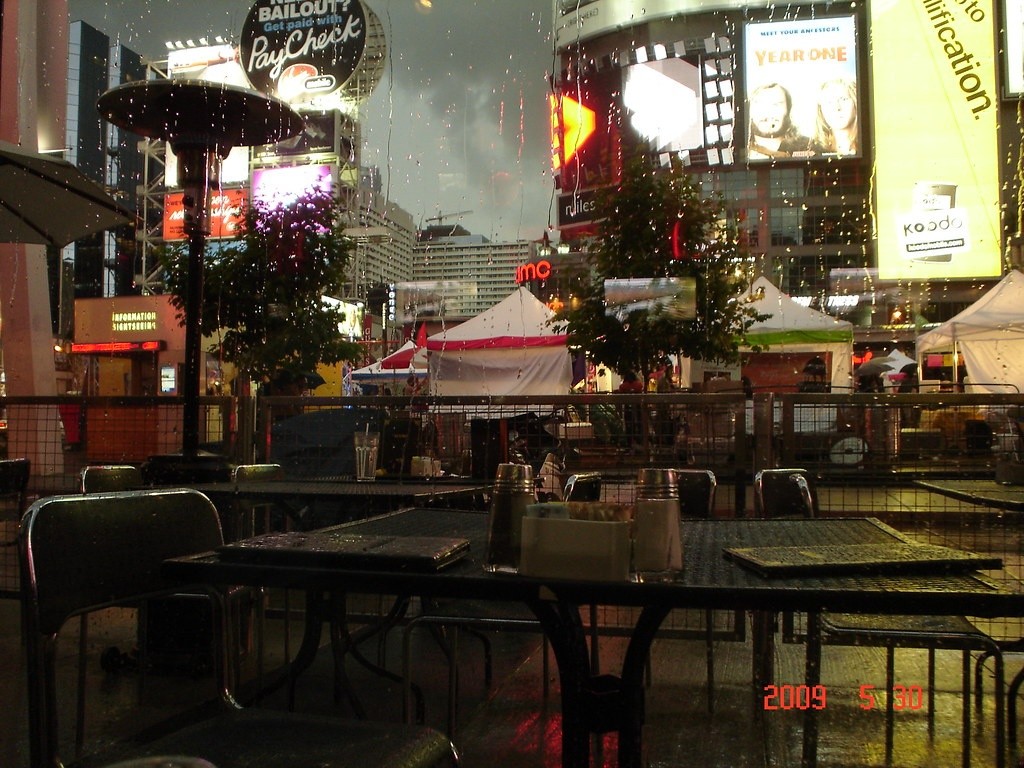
[519,512,634,581]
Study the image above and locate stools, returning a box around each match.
[822,611,1005,768]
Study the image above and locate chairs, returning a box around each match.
[16,487,462,768]
[78,465,143,497]
[562,471,601,503]
[0,458,30,647]
[753,465,811,518]
[676,468,717,518]
[234,462,283,480]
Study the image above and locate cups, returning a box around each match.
[354,432,381,482]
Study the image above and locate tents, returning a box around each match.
[680,278,853,401]
[914,269,1024,412]
[346,280,587,417]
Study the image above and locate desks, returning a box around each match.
[168,482,1024,768]
[170,479,493,653]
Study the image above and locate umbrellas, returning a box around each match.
[852,356,899,380]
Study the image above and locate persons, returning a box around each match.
[621,370,646,444]
[404,373,428,395]
[858,375,886,398]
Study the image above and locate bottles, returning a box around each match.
[481,463,534,574]
[632,469,684,582]
